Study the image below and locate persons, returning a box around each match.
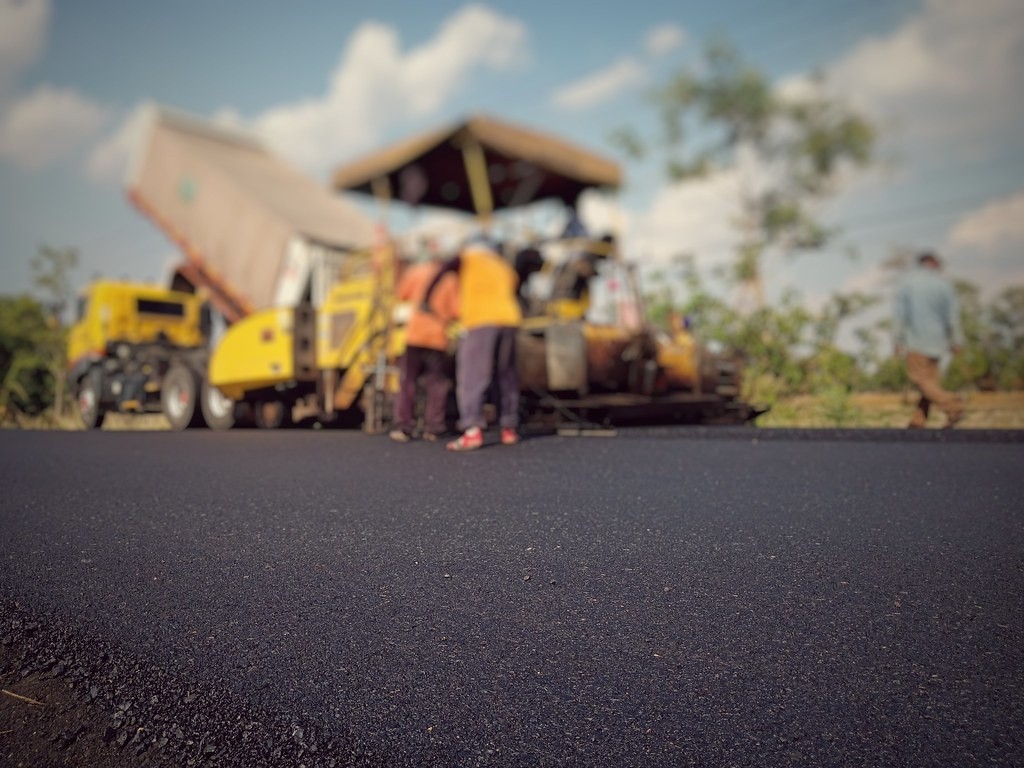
[440,231,524,452]
[889,252,968,431]
[670,313,694,354]
[387,232,462,443]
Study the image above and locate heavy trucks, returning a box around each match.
[68,108,392,430]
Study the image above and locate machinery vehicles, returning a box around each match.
[207,116,778,436]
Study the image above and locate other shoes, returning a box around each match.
[446,425,484,451]
[500,427,521,444]
